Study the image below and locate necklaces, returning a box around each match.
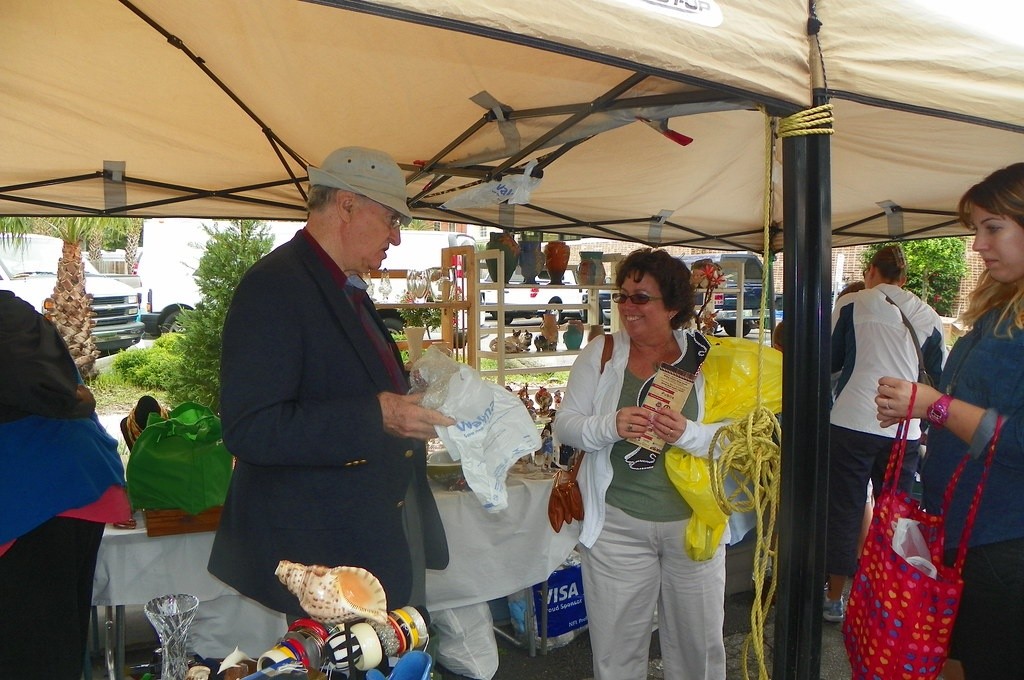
[127,402,170,445]
[363,269,392,299]
[637,345,670,372]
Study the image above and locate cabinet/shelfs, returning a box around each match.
[376,245,476,373]
[476,237,745,424]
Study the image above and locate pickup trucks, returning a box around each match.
[673,253,783,337]
[481,266,613,324]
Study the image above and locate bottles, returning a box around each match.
[432,340,451,357]
[574,251,605,285]
[485,233,570,286]
[256,621,346,672]
[587,324,606,342]
[539,314,560,342]
[563,320,585,351]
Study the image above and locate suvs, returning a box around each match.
[0,233,145,356]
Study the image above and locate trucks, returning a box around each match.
[140,218,492,348]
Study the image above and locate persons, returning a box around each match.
[875,160,1024,680]
[553,248,731,680]
[207,145,457,680]
[0,290,134,680]
[824,245,947,621]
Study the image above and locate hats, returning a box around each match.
[309,146,413,228]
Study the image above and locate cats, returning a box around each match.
[489,329,533,353]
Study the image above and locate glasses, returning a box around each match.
[354,193,401,228]
[611,292,672,305]
[862,268,869,277]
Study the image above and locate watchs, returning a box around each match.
[926,394,955,429]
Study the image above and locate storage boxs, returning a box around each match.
[142,506,224,537]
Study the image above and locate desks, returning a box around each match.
[89,480,536,676]
[521,449,777,660]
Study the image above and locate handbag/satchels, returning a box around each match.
[842,382,1001,680]
[917,371,935,388]
[547,470,584,533]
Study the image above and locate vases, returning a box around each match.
[539,313,561,352]
[562,319,585,350]
[432,340,453,356]
[515,239,545,285]
[577,249,606,285]
[404,325,427,364]
[484,231,522,285]
[544,240,571,285]
[588,325,605,343]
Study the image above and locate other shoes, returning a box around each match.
[821,593,845,620]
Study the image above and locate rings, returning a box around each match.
[627,423,632,432]
[886,399,891,409]
[668,429,674,436]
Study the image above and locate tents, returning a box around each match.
[0,0,1024,680]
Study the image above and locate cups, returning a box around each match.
[144,593,199,680]
[404,327,426,364]
